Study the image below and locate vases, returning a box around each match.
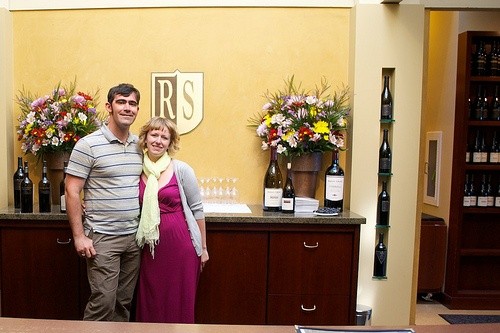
[43,151,69,205]
[288,154,322,197]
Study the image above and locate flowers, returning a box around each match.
[12,74,110,166]
[244,73,352,160]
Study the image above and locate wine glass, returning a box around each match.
[198,177,239,200]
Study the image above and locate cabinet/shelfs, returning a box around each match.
[444,31,500,310]
[0,219,360,325]
[372,119,394,280]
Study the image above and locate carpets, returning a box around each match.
[439,313,500,324]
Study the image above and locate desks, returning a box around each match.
[418,212,446,301]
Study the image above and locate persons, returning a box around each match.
[81,116,209,324]
[65,84,143,323]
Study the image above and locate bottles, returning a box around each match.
[373,232,387,277]
[281,168,297,214]
[379,129,395,173]
[262,146,283,212]
[20,161,33,213]
[323,150,348,213]
[59,161,68,213]
[381,76,393,120]
[38,160,52,213]
[378,181,392,225]
[459,43,500,209]
[13,156,25,209]
